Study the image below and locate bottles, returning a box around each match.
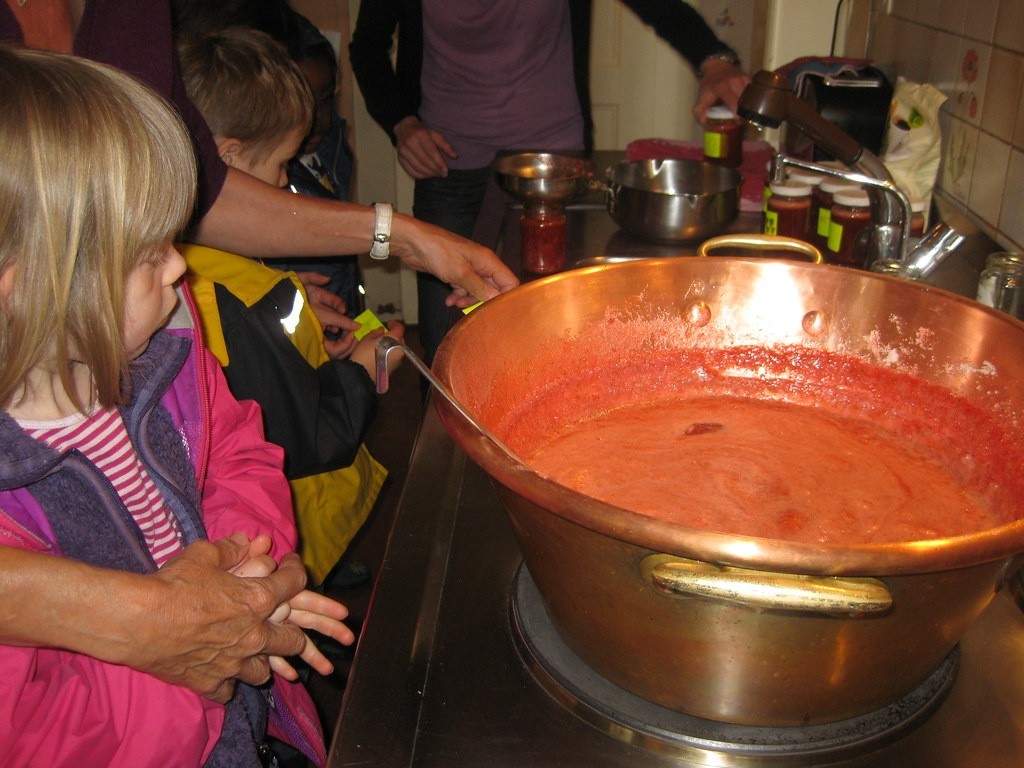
[974,251,1024,322]
[762,158,927,265]
[519,205,567,276]
[702,106,744,168]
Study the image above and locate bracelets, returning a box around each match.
[367,200,396,261]
[709,50,743,64]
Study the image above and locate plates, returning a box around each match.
[625,137,775,216]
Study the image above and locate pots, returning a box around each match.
[421,225,1024,727]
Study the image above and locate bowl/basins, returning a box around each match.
[586,158,740,247]
[490,152,597,208]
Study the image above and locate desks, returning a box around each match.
[319,149,1024,766]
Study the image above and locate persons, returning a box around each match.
[132,39,390,595]
[0,0,522,705]
[159,25,411,704]
[0,40,353,767]
[225,2,410,580]
[348,0,761,415]
